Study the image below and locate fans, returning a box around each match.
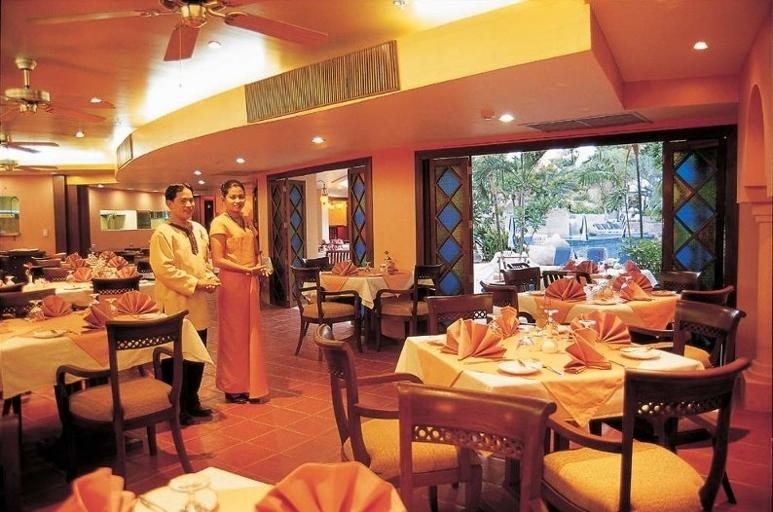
[27,0,328,61]
[0,58,116,122]
[0,134,59,153]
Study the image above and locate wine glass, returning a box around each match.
[104,298,118,317]
[364,262,371,272]
[486,308,600,356]
[563,254,633,301]
[6,242,119,287]
[28,299,45,322]
[88,293,101,314]
[168,472,210,512]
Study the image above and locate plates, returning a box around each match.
[139,313,157,319]
[33,329,65,338]
[64,287,80,290]
[651,290,676,295]
[368,273,383,277]
[497,360,542,375]
[620,347,662,360]
[530,290,545,296]
[134,485,218,512]
[595,301,616,306]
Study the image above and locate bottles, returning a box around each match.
[380,264,386,272]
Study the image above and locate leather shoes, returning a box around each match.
[180,415,193,425]
[226,392,260,404]
[188,402,213,417]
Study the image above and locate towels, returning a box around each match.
[55,467,135,512]
[253,461,408,512]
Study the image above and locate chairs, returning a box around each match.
[395,381,557,512]
[314,324,483,512]
[541,358,751,512]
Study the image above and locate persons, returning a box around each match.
[209,178,269,405]
[147,181,221,422]
